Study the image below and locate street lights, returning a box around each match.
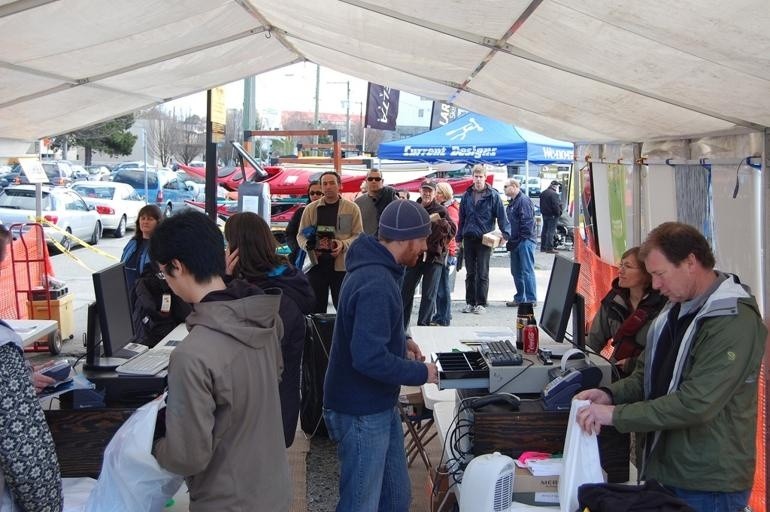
[284,72,320,158]
[328,80,351,154]
[341,101,364,148]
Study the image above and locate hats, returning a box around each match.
[379,199,433,240]
[438,182,453,199]
[420,179,435,188]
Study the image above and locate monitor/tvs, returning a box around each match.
[539,254,585,359]
[83,262,136,371]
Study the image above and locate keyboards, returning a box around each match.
[115,349,174,376]
[481,339,523,365]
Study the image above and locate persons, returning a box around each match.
[322,200,439,512]
[148,210,297,512]
[571,223,769,512]
[503,178,537,308]
[120,204,318,449]
[287,165,460,328]
[0,225,64,511]
[455,164,511,313]
[8,176,21,186]
[587,247,669,383]
[540,181,563,254]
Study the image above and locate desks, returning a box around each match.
[434,401,645,512]
[409,326,613,410]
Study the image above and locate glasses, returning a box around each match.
[368,176,381,182]
[309,191,322,196]
[157,263,168,280]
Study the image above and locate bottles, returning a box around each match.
[516,301,537,349]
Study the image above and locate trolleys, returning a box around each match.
[8,221,63,356]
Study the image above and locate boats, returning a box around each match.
[388,172,495,201]
[184,199,307,230]
[173,157,366,202]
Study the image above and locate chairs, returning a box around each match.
[398,393,438,471]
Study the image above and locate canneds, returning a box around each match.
[521,324,539,355]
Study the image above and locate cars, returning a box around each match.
[0,184,106,252]
[0,157,269,240]
[512,164,570,199]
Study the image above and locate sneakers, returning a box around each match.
[462,304,485,313]
[546,249,559,254]
[506,300,518,307]
